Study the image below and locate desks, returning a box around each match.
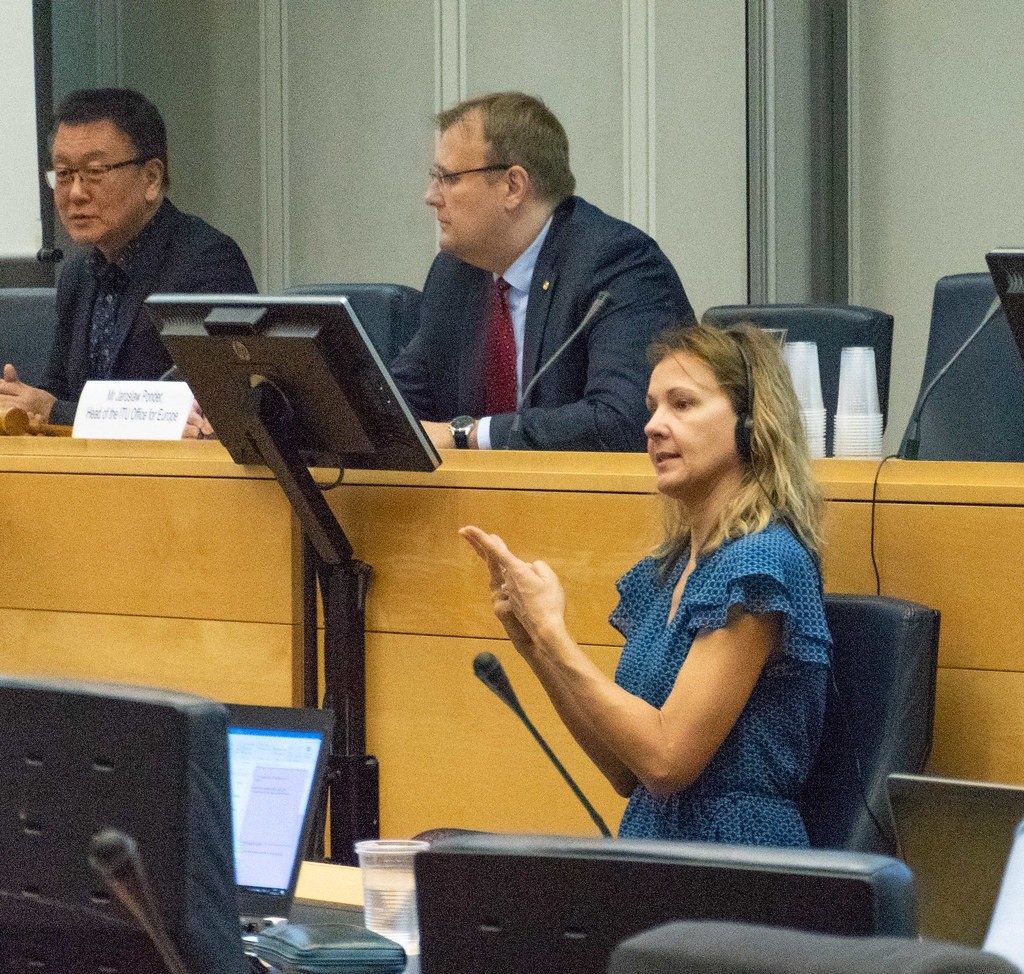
[0,434,1024,857]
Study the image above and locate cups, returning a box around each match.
[831,346,885,459]
[782,341,827,460]
[354,840,430,956]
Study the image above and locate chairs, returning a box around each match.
[901,270,1023,462]
[1,288,67,398]
[696,301,896,452]
[798,595,938,851]
[281,283,421,367]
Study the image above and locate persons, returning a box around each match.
[0,88,260,435]
[459,323,837,850]
[183,91,696,452]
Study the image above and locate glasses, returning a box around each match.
[430,163,515,194]
[44,157,155,190]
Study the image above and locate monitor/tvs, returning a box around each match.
[985,247,1024,360]
[142,291,441,473]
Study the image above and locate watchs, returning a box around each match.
[449,414,477,450]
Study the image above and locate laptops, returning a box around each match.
[885,776,1024,949]
[218,705,336,923]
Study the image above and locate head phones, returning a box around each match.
[726,332,762,462]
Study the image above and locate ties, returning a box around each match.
[479,277,519,415]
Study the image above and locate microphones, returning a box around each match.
[85,829,191,974]
[905,296,1003,462]
[473,651,617,838]
[506,289,614,451]
